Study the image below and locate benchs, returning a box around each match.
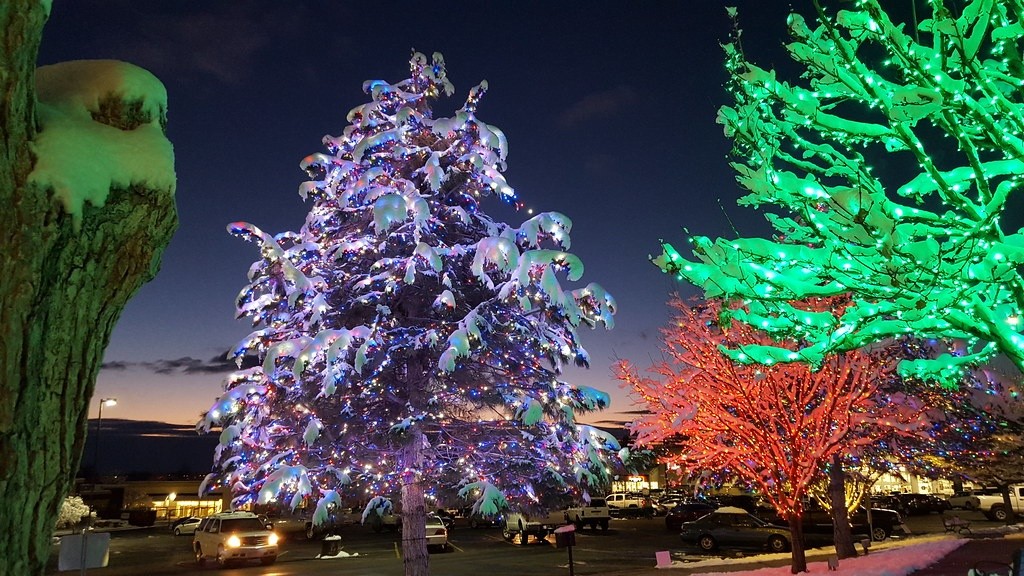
[974,548,1024,576]
[940,515,972,535]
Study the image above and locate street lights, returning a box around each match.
[86,397,118,529]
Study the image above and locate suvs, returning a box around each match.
[192,510,279,570]
[566,496,612,534]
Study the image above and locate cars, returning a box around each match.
[469,505,569,547]
[171,514,203,537]
[606,479,1024,556]
[259,501,456,553]
